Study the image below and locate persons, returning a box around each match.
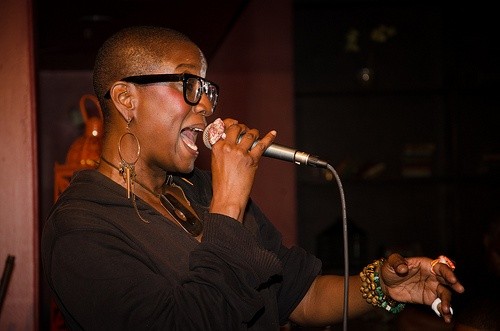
[39,25,464,331]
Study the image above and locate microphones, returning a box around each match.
[203,123,329,169]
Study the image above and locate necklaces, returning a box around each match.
[98,154,204,236]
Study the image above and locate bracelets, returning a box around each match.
[360,258,406,314]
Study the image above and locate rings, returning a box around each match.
[431,298,453,318]
[208,118,226,145]
[429,255,456,276]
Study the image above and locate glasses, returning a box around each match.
[104,72,220,112]
